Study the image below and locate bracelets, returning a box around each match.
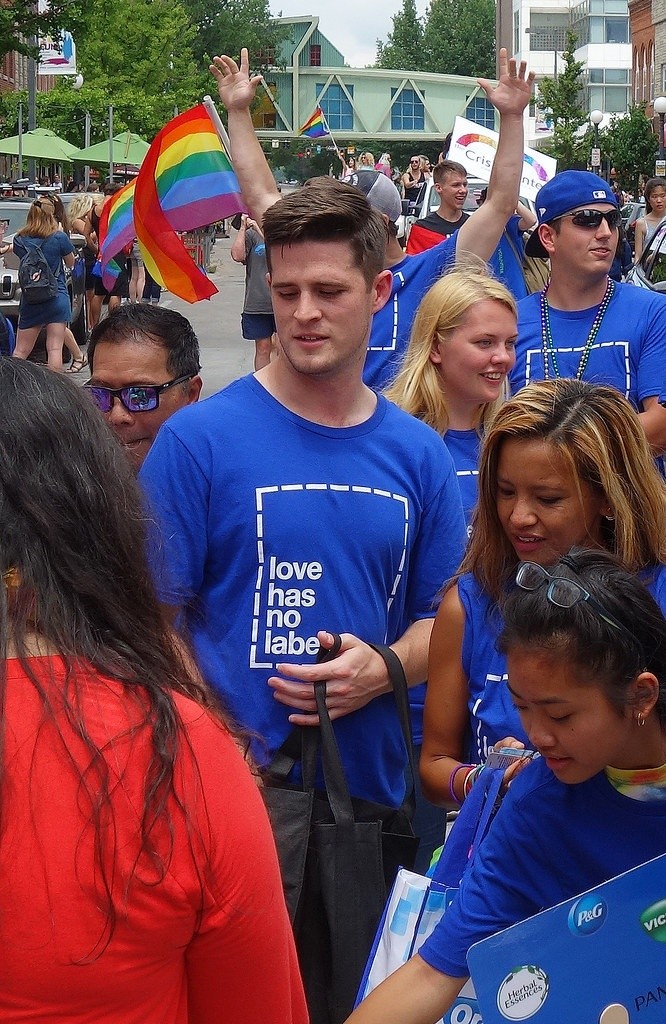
[464,768,477,799]
[479,767,486,776]
[462,766,475,796]
[473,764,484,786]
[450,764,472,807]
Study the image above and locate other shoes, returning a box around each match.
[85,326,94,342]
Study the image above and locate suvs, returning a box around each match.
[0,192,105,364]
[401,175,540,260]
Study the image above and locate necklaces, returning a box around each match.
[544,279,614,381]
[540,273,611,381]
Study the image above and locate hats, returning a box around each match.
[338,170,403,222]
[524,169,618,259]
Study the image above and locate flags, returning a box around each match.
[92,176,137,294]
[298,108,329,138]
[133,103,249,304]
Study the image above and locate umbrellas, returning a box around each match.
[67,132,150,186]
[0,128,81,185]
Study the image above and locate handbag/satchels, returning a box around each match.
[502,229,551,295]
[238,627,419,1024]
[354,767,488,1024]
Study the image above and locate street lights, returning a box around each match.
[591,109,603,174]
[654,96,666,160]
[525,28,557,146]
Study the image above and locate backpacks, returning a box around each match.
[18,233,59,305]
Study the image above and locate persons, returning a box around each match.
[0,49,666,1024]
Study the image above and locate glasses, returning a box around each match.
[361,156,366,159]
[80,373,197,413]
[547,208,623,228]
[516,560,643,654]
[410,160,419,165]
[476,199,483,205]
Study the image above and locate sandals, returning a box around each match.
[66,354,88,374]
